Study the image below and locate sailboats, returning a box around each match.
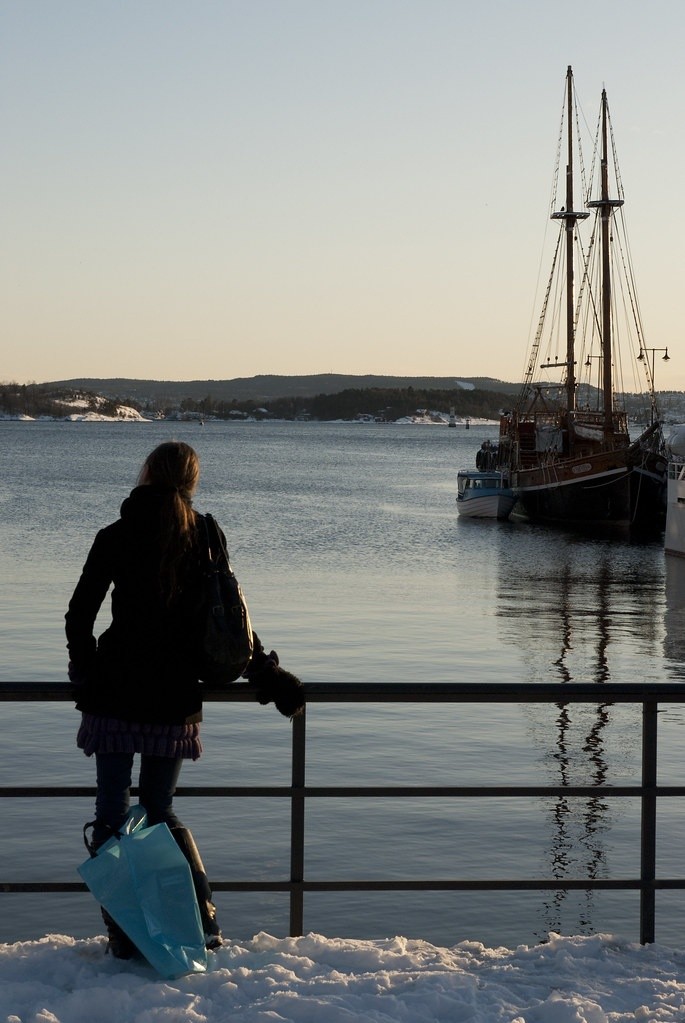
[497,62,670,549]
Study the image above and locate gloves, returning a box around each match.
[255,666,306,718]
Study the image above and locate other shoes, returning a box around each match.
[107,939,134,960]
[202,923,224,950]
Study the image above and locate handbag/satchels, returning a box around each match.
[199,513,252,685]
[76,806,208,981]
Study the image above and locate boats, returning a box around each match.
[455,470,515,521]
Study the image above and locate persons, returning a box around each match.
[65,441,256,962]
